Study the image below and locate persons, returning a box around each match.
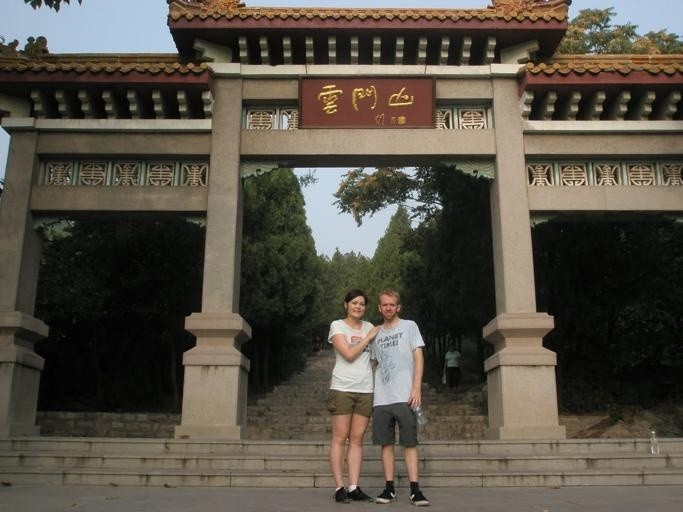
[443,343,464,389]
[328,288,381,503]
[370,288,430,506]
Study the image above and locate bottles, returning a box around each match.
[650,430,659,455]
[409,403,428,425]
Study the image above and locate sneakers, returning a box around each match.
[334,487,349,504]
[377,488,396,503]
[409,491,430,506]
[349,487,372,503]
